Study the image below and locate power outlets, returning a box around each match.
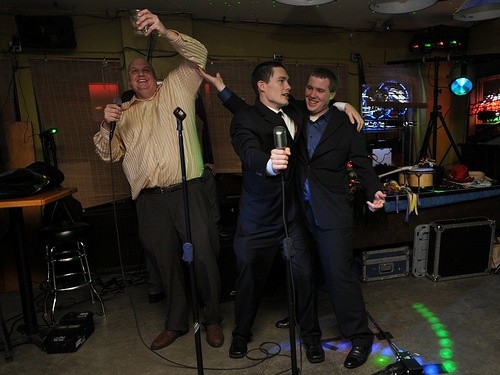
[352,52,361,62]
[274,53,283,60]
[9,40,22,53]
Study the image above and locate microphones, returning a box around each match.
[110,96,122,132]
[273,125,287,150]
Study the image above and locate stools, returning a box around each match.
[43,222,106,325]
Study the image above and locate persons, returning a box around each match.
[91,6,225,352]
[191,65,388,369]
[228,58,364,364]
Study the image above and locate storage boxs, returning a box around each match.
[425,216,497,282]
[360,245,410,281]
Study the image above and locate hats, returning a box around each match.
[448,163,472,181]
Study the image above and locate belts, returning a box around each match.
[140,177,207,195]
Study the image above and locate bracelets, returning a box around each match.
[158,26,169,40]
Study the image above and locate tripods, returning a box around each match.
[416,57,468,167]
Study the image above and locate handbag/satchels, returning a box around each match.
[0,160,65,199]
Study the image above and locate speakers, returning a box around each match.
[15,14,78,52]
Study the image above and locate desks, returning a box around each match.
[0,187,79,350]
[352,183,500,248]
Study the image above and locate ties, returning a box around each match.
[277,111,294,148]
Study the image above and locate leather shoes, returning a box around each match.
[148,327,188,349]
[275,316,299,329]
[344,345,372,368]
[229,335,251,357]
[206,324,224,347]
[303,340,325,364]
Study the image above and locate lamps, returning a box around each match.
[368,0,437,14]
[446,61,476,96]
[453,0,500,22]
[410,37,458,51]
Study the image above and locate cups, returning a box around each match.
[453,165,468,180]
